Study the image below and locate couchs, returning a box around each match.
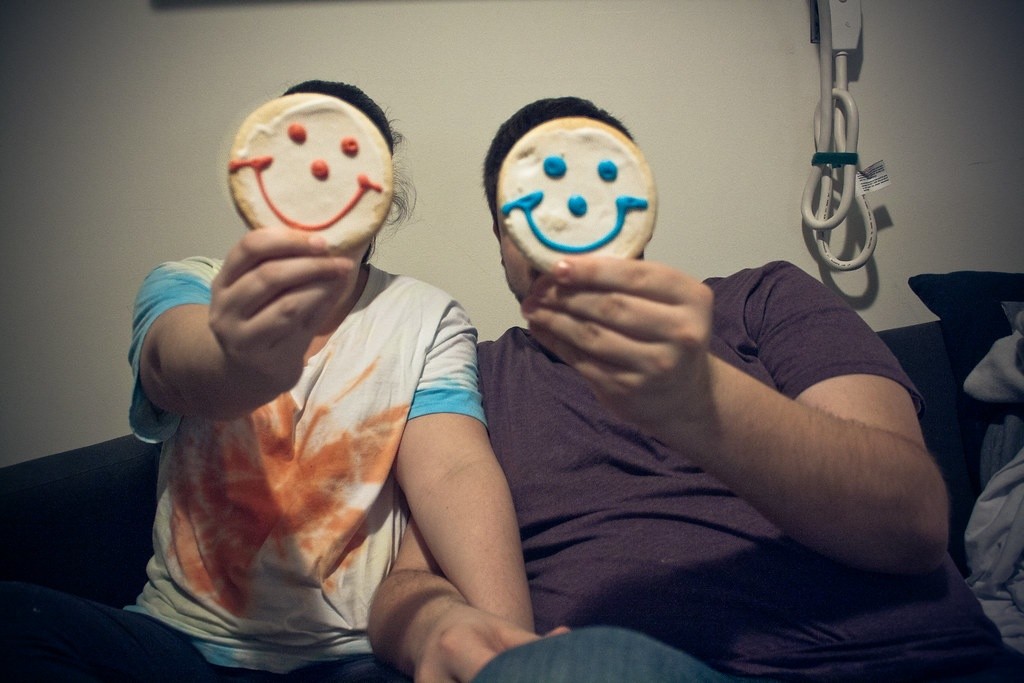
[0,319,972,683]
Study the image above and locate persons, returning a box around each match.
[367,97,1024,683]
[0,80,536,683]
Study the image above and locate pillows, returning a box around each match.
[907,271,1024,484]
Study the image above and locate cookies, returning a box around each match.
[228,93,394,255]
[496,117,658,276]
